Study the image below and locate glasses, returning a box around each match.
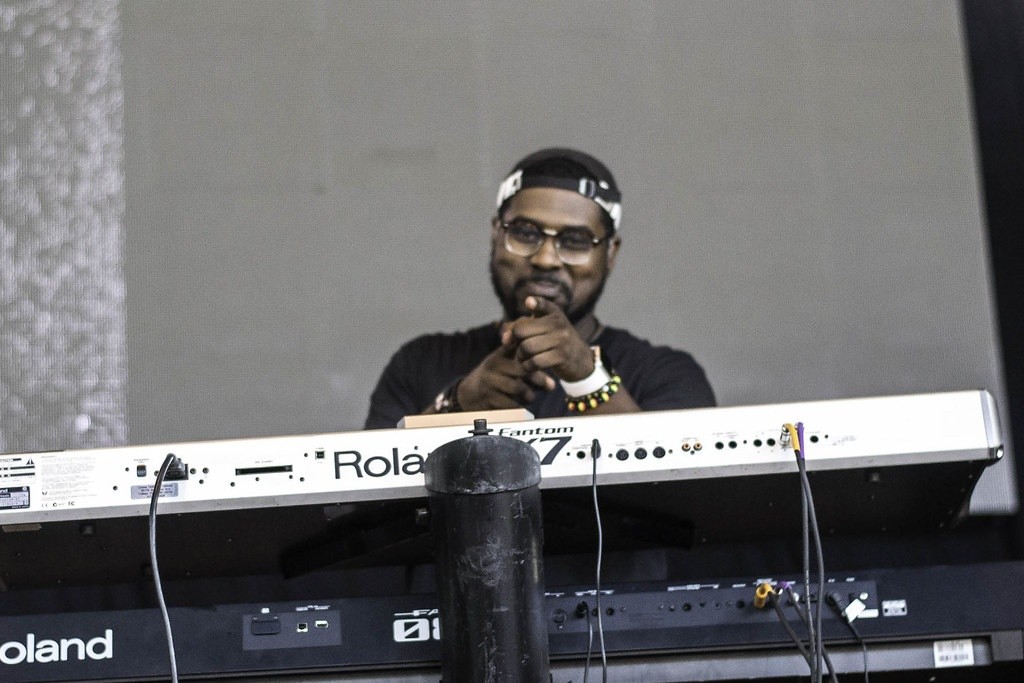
[499,218,611,266]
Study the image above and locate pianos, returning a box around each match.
[0,389,1003,612]
[0,556,1023,682]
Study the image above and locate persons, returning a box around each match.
[362,147,717,431]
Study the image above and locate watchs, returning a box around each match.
[560,345,612,397]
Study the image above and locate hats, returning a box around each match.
[496,148,622,232]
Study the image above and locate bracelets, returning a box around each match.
[433,379,464,413]
[564,376,621,412]
